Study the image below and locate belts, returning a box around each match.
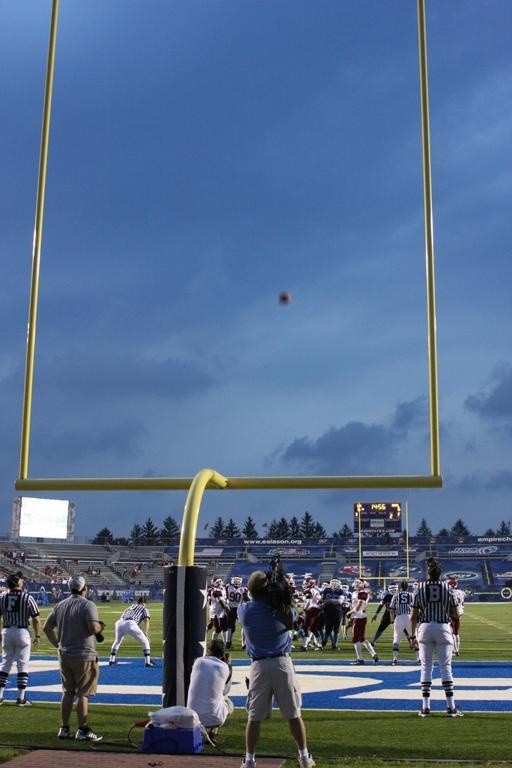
[251,652,289,663]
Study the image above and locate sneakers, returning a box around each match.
[351,658,364,665]
[292,631,349,652]
[445,705,464,717]
[108,660,118,666]
[206,733,224,745]
[74,728,105,743]
[417,705,431,717]
[369,641,376,646]
[15,697,33,707]
[57,727,76,740]
[240,758,256,768]
[373,654,380,664]
[298,753,316,768]
[391,658,397,666]
[144,660,159,668]
[417,659,421,665]
[0,698,4,705]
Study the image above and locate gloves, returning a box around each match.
[345,607,357,619]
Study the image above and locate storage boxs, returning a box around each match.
[143,726,203,753]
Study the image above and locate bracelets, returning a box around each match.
[409,635,416,640]
[35,634,41,638]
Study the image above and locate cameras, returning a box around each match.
[93,621,105,643]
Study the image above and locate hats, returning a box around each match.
[400,578,408,590]
[138,597,147,604]
[246,570,269,601]
[7,571,24,588]
[427,558,441,578]
[67,574,87,592]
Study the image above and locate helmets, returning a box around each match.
[443,576,458,588]
[230,575,244,586]
[283,573,297,593]
[210,575,224,588]
[387,581,398,595]
[302,577,316,594]
[351,579,366,591]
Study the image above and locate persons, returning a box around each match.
[106,596,160,670]
[184,638,235,746]
[128,559,212,578]
[3,547,30,565]
[1,570,44,709]
[86,564,102,577]
[234,569,318,768]
[407,561,465,719]
[44,564,68,603]
[42,572,104,742]
[205,571,467,669]
[0,575,7,582]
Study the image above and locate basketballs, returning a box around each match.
[280,292,291,305]
